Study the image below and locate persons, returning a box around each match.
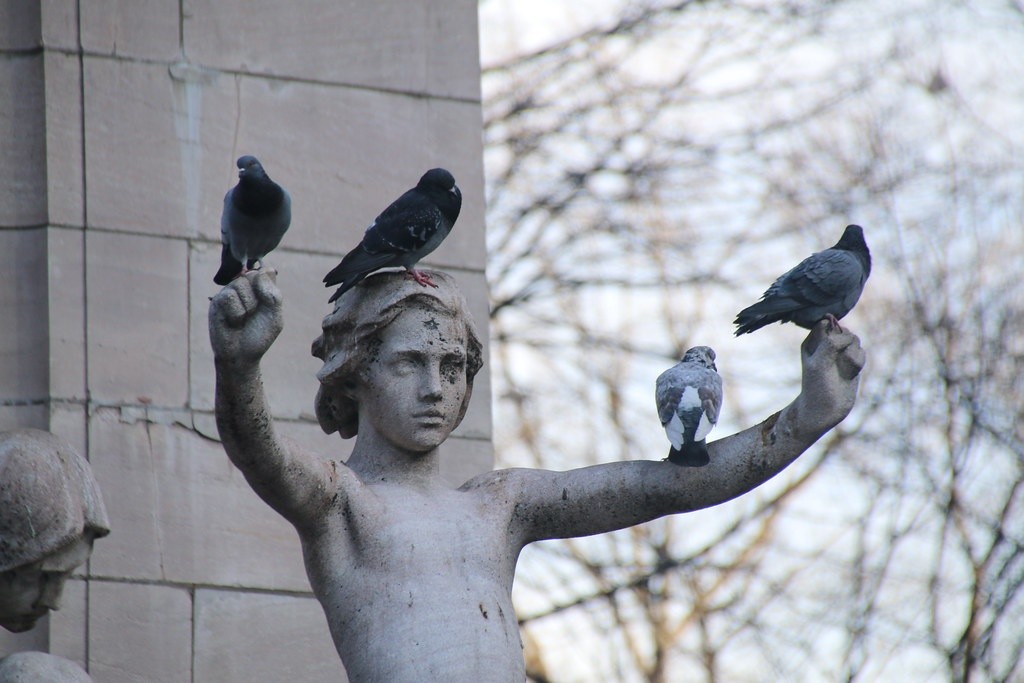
[1,428,111,681]
[210,268,867,683]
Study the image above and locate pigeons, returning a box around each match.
[734,224,872,337]
[322,168,462,303]
[212,154,292,286]
[654,345,723,467]
